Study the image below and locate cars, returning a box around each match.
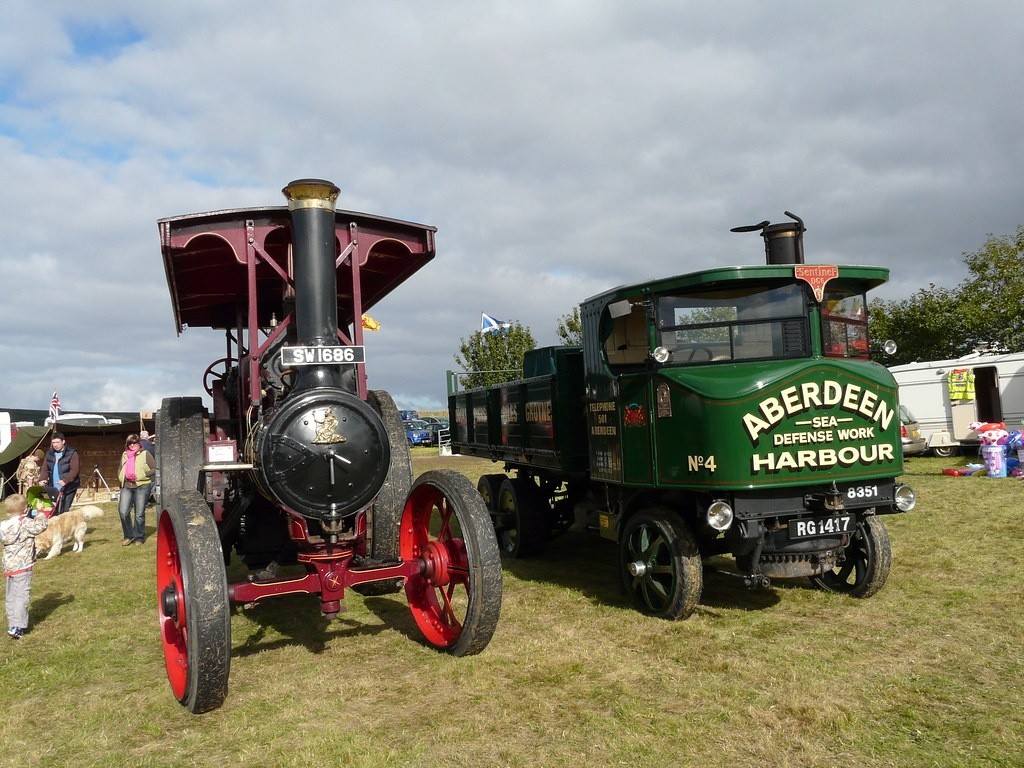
[400,409,450,446]
[897,404,927,456]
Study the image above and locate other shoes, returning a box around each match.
[7,626,25,638]
[122,537,134,546]
[134,541,142,546]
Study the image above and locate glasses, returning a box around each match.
[128,441,138,445]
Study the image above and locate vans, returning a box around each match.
[882,350,1024,457]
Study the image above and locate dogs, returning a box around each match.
[27,504,104,562]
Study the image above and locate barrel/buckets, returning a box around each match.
[981,445,1007,478]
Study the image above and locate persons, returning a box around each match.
[37,432,81,515]
[0,493,48,640]
[116,435,156,547]
[139,431,156,509]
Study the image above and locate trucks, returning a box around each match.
[447,211,917,623]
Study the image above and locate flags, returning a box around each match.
[362,314,381,331]
[48,391,61,419]
[482,313,512,337]
